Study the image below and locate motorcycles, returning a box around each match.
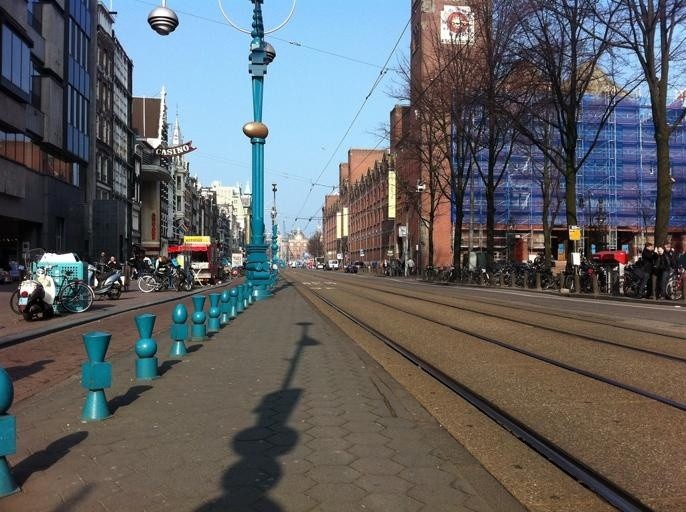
[87,264,124,300]
[17,265,58,322]
[422,252,686,302]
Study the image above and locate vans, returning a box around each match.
[326,259,339,271]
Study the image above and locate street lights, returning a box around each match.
[242,1,276,304]
[271,183,278,235]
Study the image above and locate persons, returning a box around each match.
[382,257,389,273]
[657,243,671,300]
[663,241,678,277]
[637,241,659,299]
[406,257,416,276]
[97,248,179,282]
[371,257,377,274]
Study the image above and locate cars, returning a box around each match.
[345,260,366,273]
[316,264,324,269]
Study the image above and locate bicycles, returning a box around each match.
[137,265,194,293]
[9,264,95,313]
